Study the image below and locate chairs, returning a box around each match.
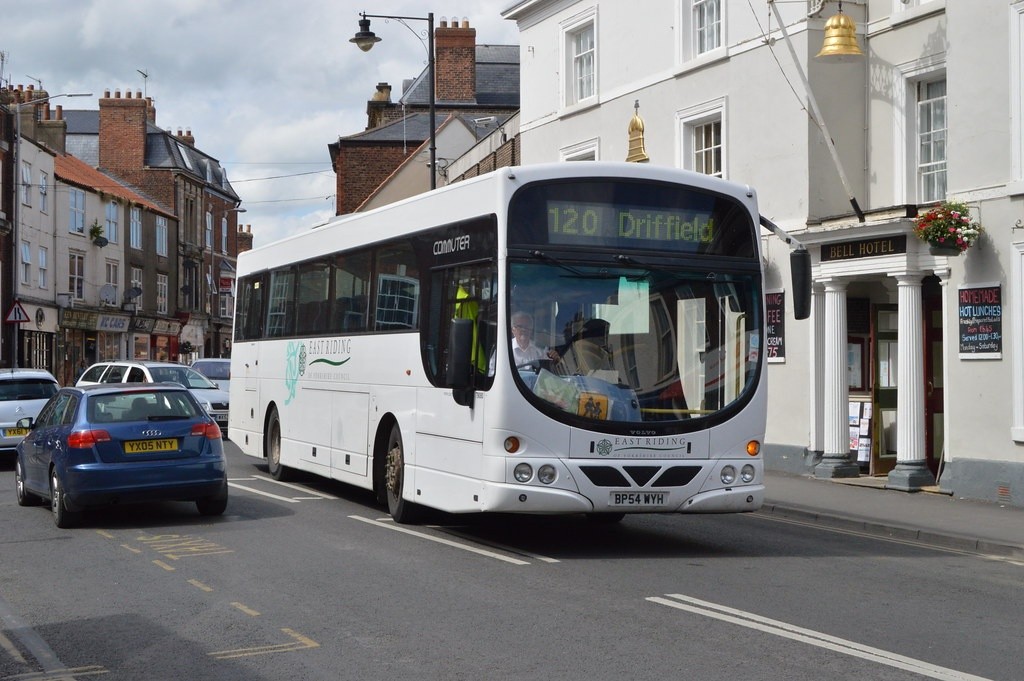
[127,397,163,419]
[95,403,113,421]
[269,290,419,338]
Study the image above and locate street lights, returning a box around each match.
[348,10,435,190]
[209,207,245,358]
[13,91,94,366]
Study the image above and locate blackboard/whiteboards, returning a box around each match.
[958,282,1004,361]
[765,289,787,365]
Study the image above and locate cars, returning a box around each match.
[14,382,229,529]
[0,366,62,450]
[186,357,231,398]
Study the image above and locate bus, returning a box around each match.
[228,160,812,531]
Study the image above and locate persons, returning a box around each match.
[488,310,559,380]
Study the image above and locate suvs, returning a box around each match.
[76,359,230,439]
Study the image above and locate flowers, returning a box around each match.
[912,202,979,251]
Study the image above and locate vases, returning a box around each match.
[928,237,961,256]
